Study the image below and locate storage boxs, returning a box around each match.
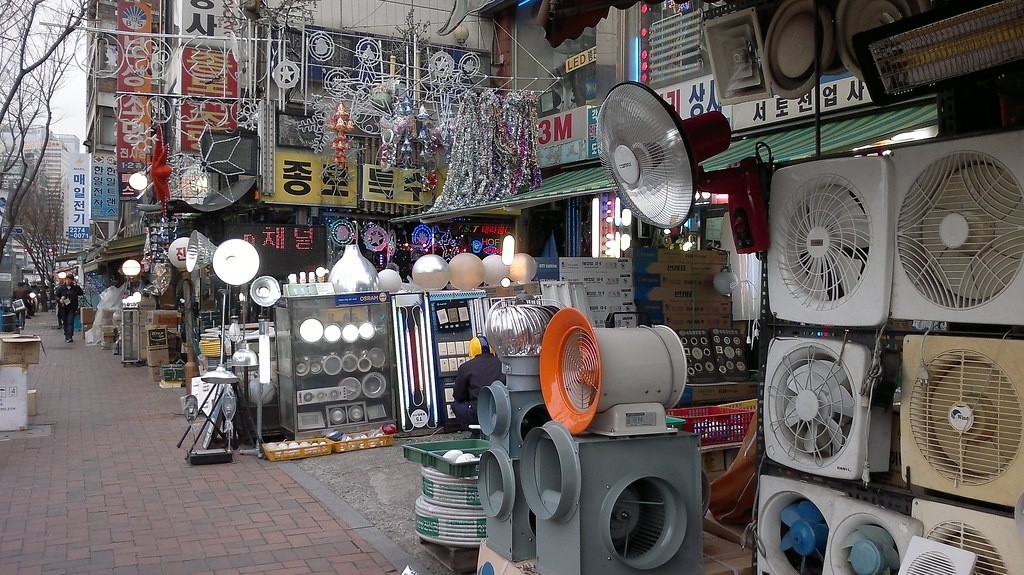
[81,307,95,323]
[148,366,162,382]
[0,364,28,431]
[145,325,169,350]
[82,324,93,338]
[0,334,47,365]
[146,348,170,367]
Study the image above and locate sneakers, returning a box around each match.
[463,431,480,439]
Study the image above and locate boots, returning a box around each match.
[21,322,24,330]
[16,324,20,327]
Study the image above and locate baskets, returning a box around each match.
[667,405,756,447]
[324,428,399,454]
[261,437,334,462]
[717,398,758,411]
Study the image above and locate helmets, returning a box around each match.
[468,335,496,360]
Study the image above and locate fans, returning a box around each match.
[911,497,1024,575]
[900,334,1024,507]
[594,81,772,255]
[889,131,1024,326]
[821,495,924,575]
[764,337,894,479]
[767,156,893,328]
[757,475,848,575]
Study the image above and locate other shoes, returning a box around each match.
[57,324,62,329]
[25,316,31,319]
[64,335,73,343]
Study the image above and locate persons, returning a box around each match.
[12,280,65,330]
[451,336,507,439]
[56,274,84,343]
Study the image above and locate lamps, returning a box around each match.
[232,340,259,367]
[250,276,281,308]
[186,230,217,273]
[202,290,239,384]
[212,239,261,286]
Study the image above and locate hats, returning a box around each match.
[65,274,74,281]
[17,282,26,287]
